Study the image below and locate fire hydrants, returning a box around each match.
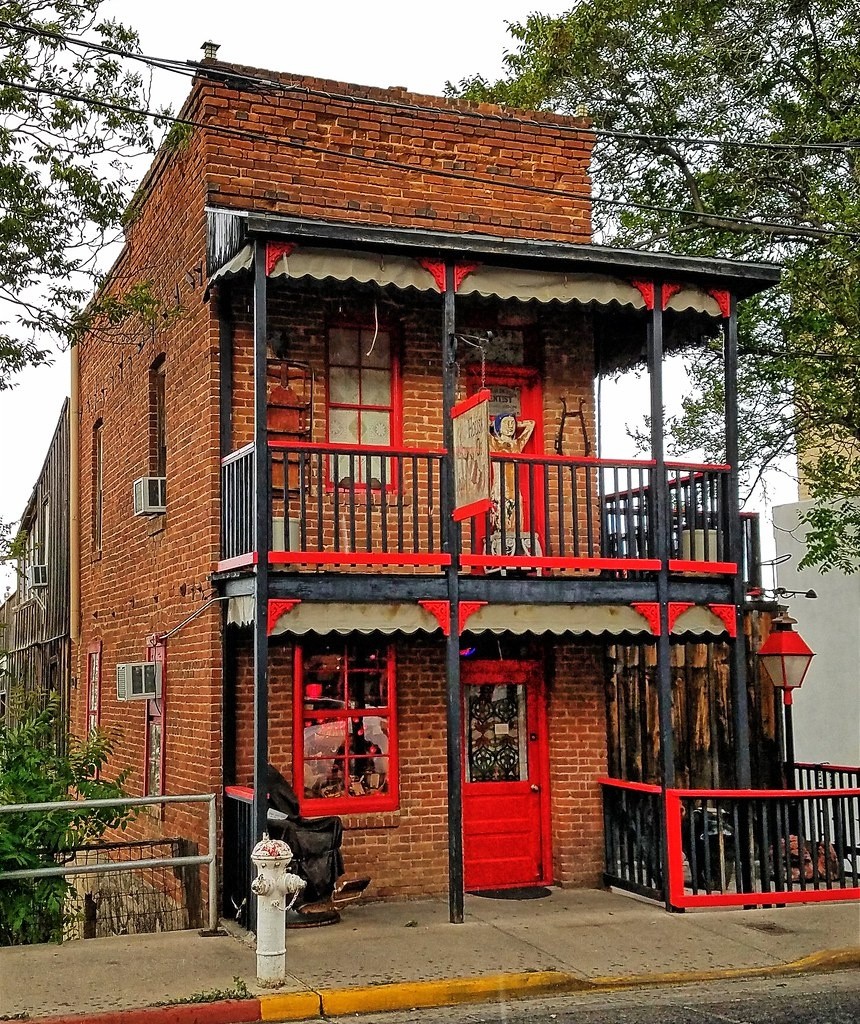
[246,832,309,989]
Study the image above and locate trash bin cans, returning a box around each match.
[271,516,302,551]
[682,529,722,576]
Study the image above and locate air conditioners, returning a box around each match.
[117,662,156,701]
[132,476,166,517]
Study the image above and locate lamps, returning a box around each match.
[756,611,817,786]
